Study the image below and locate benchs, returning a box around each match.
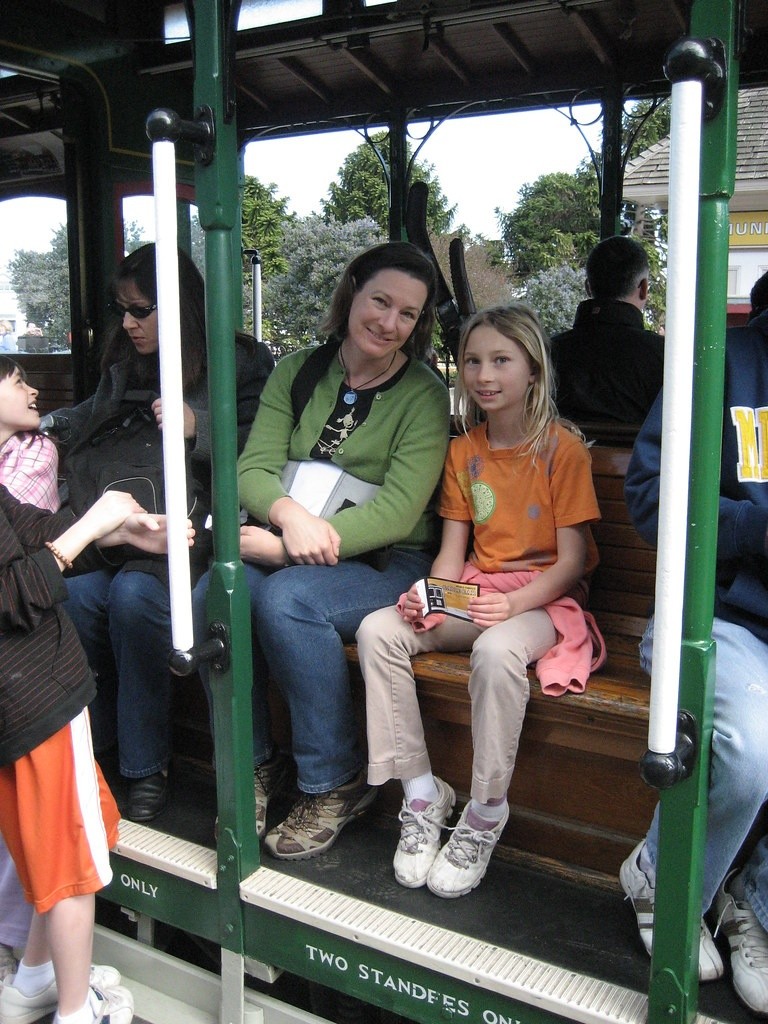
[263,444,662,903]
[0,352,76,418]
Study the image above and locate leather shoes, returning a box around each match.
[95,729,118,762]
[126,761,171,820]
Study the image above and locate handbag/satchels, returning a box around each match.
[64,425,214,590]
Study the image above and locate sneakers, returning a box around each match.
[263,769,380,861]
[50,985,134,1024]
[0,963,122,1024]
[392,776,458,889]
[215,749,289,845]
[618,839,724,982]
[425,799,510,899]
[714,866,768,1012]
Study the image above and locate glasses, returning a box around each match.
[106,297,157,319]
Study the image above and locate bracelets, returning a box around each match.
[46,542,74,568]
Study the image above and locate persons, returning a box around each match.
[46,244,274,821]
[620,272,768,1013]
[0,355,196,1024]
[358,306,602,897]
[190,242,451,861]
[541,236,665,449]
